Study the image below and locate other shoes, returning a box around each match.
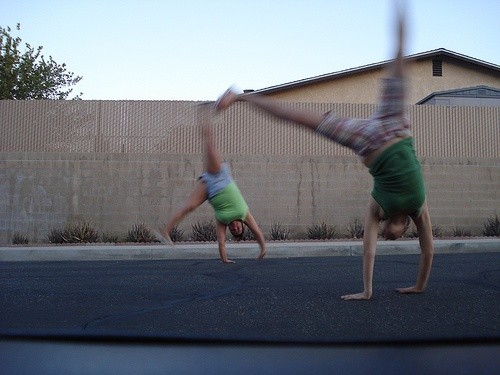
[154,230,177,249]
[212,85,244,113]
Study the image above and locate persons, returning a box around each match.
[214,0,435,301]
[153,98,268,264]
